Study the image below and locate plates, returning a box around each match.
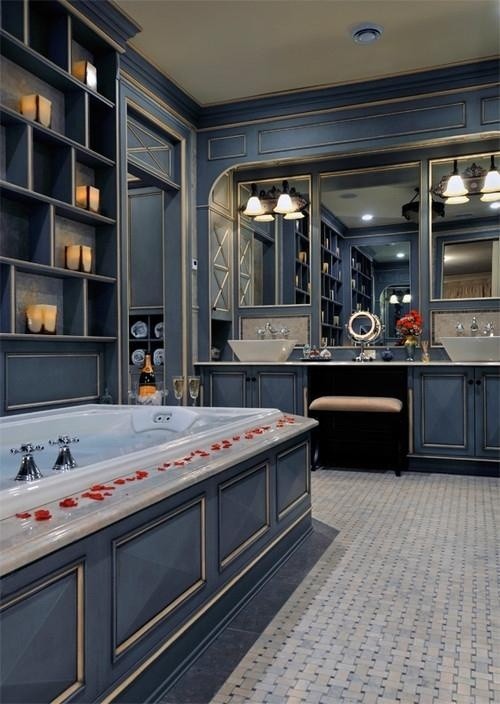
[132,349,145,365]
[131,320,148,338]
[154,322,163,338]
[154,348,164,366]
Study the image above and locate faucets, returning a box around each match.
[266,322,279,337]
[471,317,479,332]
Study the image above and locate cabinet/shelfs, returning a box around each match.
[282,208,375,345]
[407,365,500,462]
[125,179,164,405]
[194,364,306,416]
[1,0,145,418]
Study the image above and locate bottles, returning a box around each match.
[303,343,332,360]
[138,354,156,397]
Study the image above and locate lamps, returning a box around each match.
[401,186,445,225]
[236,179,310,216]
[386,285,411,308]
[429,154,500,198]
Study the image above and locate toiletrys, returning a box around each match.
[310,344,320,359]
[319,347,332,359]
[302,343,311,359]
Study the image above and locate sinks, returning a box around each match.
[439,336,500,362]
[227,339,298,362]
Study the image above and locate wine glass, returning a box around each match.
[187,375,201,406]
[171,375,185,405]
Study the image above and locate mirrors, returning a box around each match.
[238,172,312,308]
[318,159,424,350]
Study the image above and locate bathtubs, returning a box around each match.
[0,403,284,522]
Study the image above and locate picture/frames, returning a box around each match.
[427,150,500,303]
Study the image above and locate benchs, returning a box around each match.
[308,394,404,476]
